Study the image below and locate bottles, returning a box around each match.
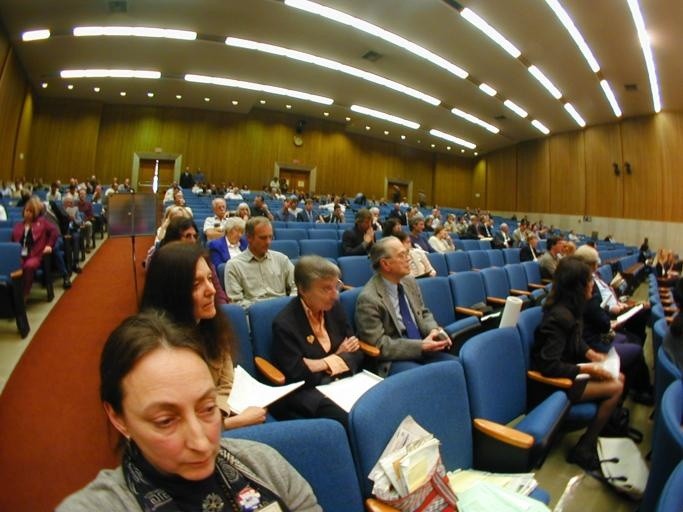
[215,223,222,231]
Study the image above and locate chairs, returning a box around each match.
[0,184,108,339]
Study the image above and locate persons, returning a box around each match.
[52,304,324,511]
[136,166,682,472]
[1,172,134,311]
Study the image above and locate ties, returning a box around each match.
[398,284,421,339]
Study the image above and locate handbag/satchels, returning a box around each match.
[597,437,649,498]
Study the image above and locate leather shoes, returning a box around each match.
[85,244,90,253]
[62,274,71,288]
[73,263,82,274]
[630,379,655,405]
[624,427,643,442]
[566,448,595,471]
